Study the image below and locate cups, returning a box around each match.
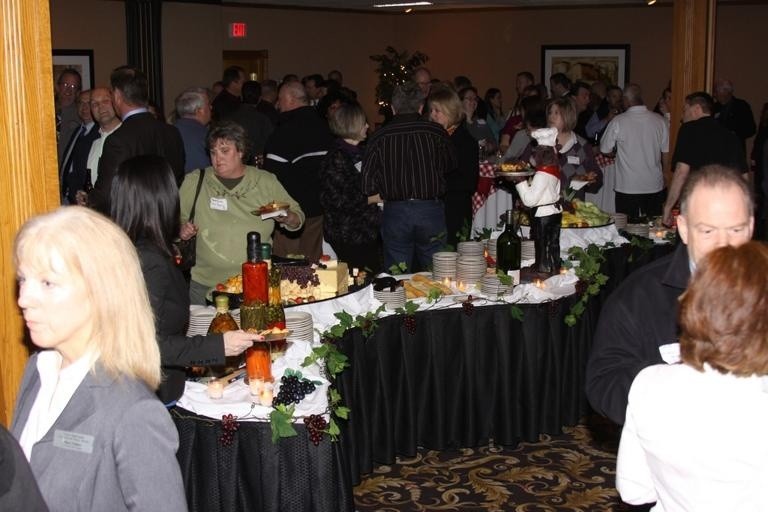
[253,156,266,172]
[248,375,265,397]
[206,378,223,400]
[260,387,273,407]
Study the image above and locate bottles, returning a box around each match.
[592,132,599,156]
[241,232,270,307]
[261,244,273,272]
[496,209,522,286]
[206,295,240,333]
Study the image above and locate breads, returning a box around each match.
[280,260,349,300]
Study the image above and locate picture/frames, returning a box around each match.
[53,48,96,111]
[540,43,632,104]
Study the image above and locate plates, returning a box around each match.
[373,285,408,308]
[206,263,378,309]
[482,238,496,262]
[186,303,241,337]
[456,241,487,283]
[608,213,628,230]
[480,275,514,295]
[623,222,650,239]
[255,328,296,344]
[522,240,536,260]
[432,252,461,280]
[499,205,617,230]
[283,311,314,347]
[259,199,290,215]
[495,170,537,177]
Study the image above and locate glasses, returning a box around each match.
[464,96,479,102]
[64,82,80,88]
[417,82,432,87]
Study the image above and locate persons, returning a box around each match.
[54,66,767,312]
[584,164,767,510]
[107,153,264,411]
[0,206,186,511]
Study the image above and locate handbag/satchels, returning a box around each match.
[172,235,196,267]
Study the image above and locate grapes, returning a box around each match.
[269,374,315,407]
[319,338,339,351]
[548,300,560,319]
[462,300,474,317]
[401,314,418,337]
[274,263,320,288]
[576,281,588,298]
[621,243,631,257]
[303,415,325,446]
[218,414,241,447]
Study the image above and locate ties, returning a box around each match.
[61,127,87,198]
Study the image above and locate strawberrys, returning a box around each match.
[320,255,329,260]
